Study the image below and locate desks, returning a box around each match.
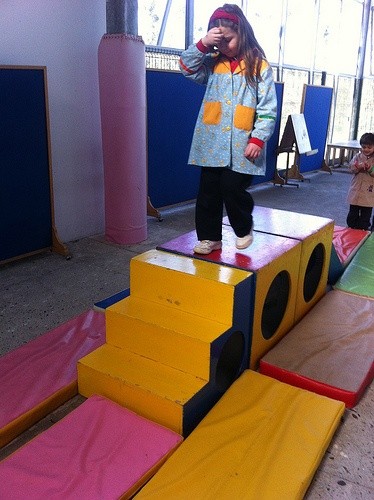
[326,140,363,168]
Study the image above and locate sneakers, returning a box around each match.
[193,240,222,254]
[236,228,253,249]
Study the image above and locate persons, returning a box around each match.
[178,4,278,254]
[347,133,374,231]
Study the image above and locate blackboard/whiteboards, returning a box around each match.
[280,114,312,154]
